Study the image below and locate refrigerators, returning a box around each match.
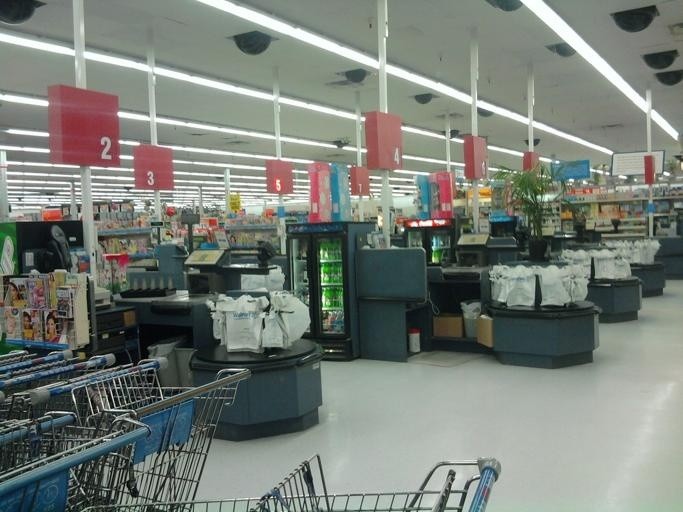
[284,221,376,362]
[404,226,458,267]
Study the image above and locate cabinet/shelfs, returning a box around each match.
[474,194,683,238]
[96,226,157,261]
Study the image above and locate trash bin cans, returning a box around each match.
[460,301,481,338]
[147,338,196,386]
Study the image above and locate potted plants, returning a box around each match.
[499,162,570,261]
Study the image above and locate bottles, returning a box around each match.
[323,311,344,333]
[301,238,342,310]
[431,233,440,264]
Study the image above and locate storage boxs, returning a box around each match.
[432,312,493,348]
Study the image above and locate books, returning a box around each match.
[5,271,73,347]
